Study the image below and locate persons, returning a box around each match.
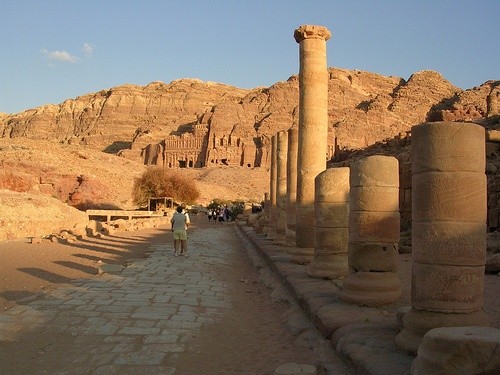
[207,204,262,226]
[170,205,189,258]
[171,202,191,256]
